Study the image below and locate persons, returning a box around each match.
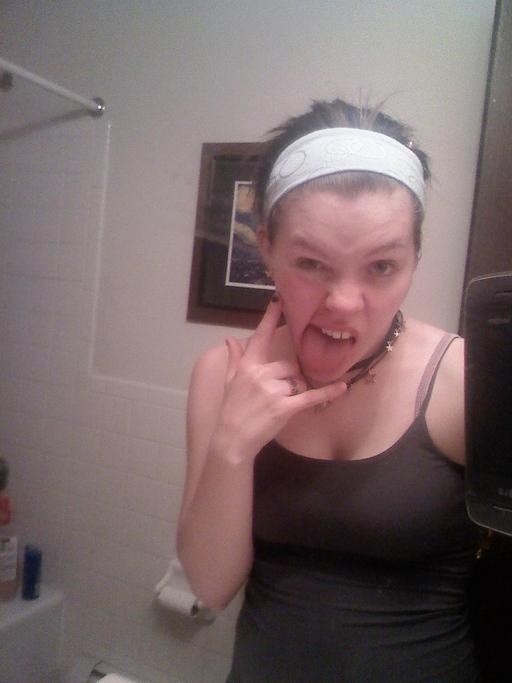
[179,99,483,682]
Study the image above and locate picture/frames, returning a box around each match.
[184,140,296,332]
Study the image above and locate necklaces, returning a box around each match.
[292,307,410,415]
